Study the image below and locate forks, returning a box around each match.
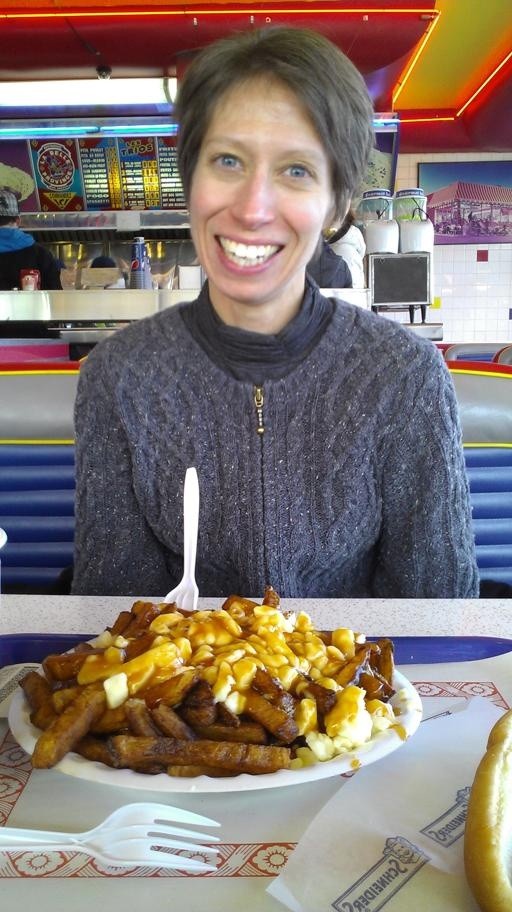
[165,466,200,610]
[0,803,224,873]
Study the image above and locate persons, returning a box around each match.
[324,208,369,292]
[72,24,480,597]
[307,232,354,290]
[1,189,63,290]
[91,256,125,288]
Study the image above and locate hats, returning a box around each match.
[0,190,19,217]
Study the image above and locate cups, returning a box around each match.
[130,236,153,288]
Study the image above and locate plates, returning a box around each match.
[8,642,423,793]
